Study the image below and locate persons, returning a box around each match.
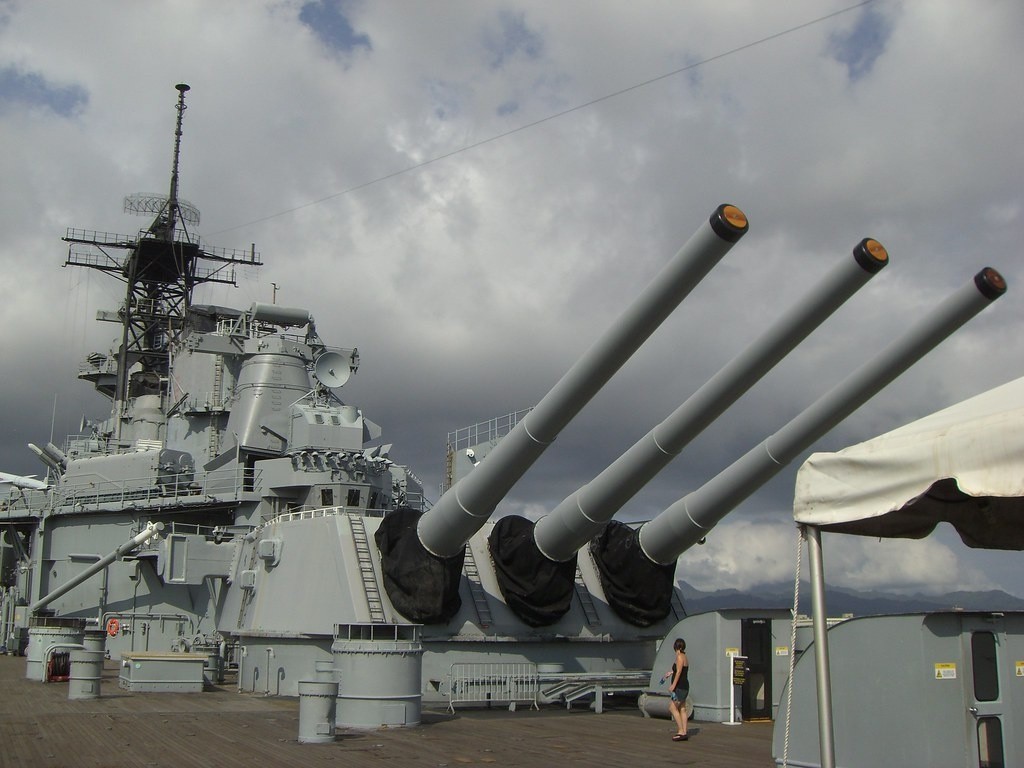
[665,638,689,741]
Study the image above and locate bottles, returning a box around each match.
[657,674,668,686]
[668,690,677,702]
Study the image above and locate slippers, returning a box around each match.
[672,734,689,741]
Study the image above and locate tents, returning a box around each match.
[793,373,1024,768]
